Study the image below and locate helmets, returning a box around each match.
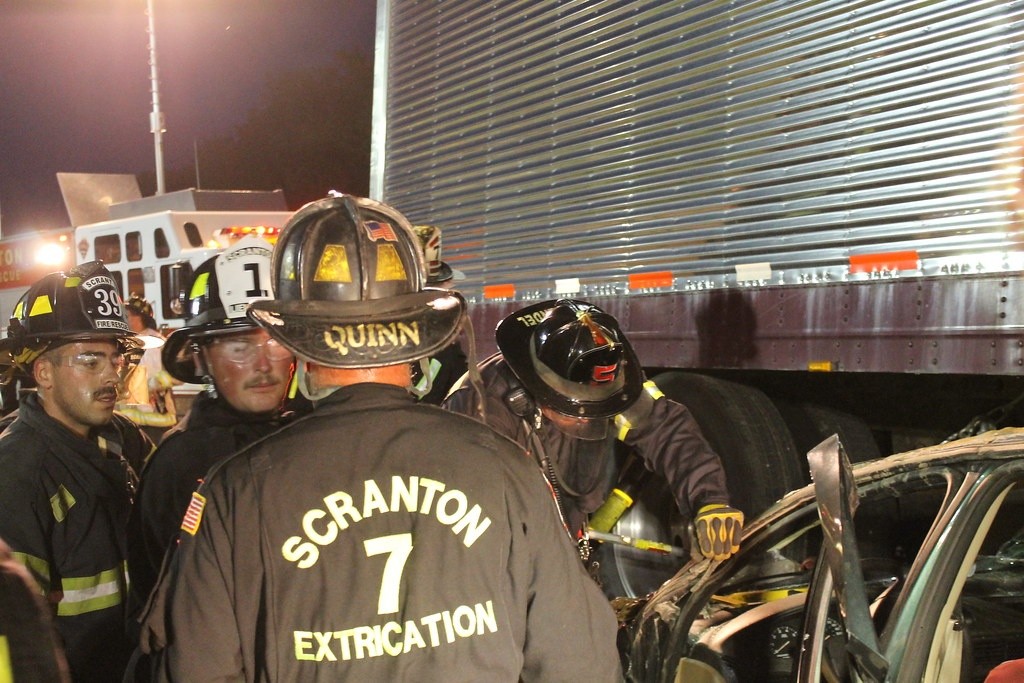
[245,190,466,370]
[159,237,279,384]
[414,223,453,283]
[0,258,144,377]
[494,298,645,441]
[122,296,159,332]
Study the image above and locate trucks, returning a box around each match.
[372,0,1024,597]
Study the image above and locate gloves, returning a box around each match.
[694,503,744,560]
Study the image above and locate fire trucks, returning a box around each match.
[0,171,296,337]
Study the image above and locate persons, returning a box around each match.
[439,298,745,627]
[0,259,180,683]
[127,190,623,683]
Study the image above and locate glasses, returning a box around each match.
[199,335,295,365]
[45,352,131,377]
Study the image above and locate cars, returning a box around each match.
[616,422,1023,683]
